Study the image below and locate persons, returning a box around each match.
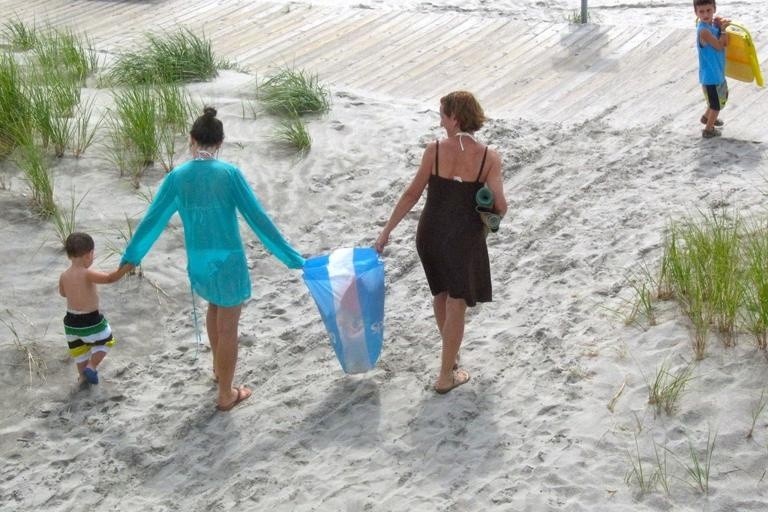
[694,0,731,137]
[58,232,134,387]
[120,107,297,405]
[377,92,506,396]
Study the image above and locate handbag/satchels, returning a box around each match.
[304,248,385,374]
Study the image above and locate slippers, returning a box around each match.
[434,368,469,394]
[453,347,459,370]
[79,381,89,393]
[211,371,219,383]
[83,368,98,385]
[216,387,252,411]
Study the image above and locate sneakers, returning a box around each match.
[700,115,724,126]
[702,127,721,138]
